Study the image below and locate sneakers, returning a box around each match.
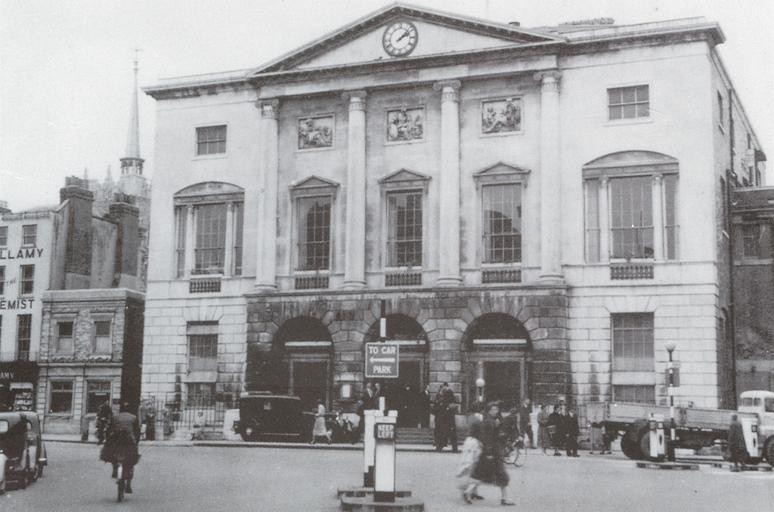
[96,437,202,494]
[517,444,612,457]
[731,462,745,472]
[434,442,459,451]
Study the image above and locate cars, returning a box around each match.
[1,410,48,489]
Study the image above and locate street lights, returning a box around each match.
[664,340,678,450]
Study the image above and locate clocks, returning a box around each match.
[383,21,418,58]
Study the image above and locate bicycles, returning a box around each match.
[110,457,130,503]
[499,435,529,467]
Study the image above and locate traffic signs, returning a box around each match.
[365,343,399,379]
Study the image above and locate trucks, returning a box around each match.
[588,388,774,471]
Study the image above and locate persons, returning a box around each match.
[95,414,105,446]
[106,402,140,493]
[161,401,175,441]
[457,400,487,499]
[145,407,157,442]
[463,400,517,506]
[190,409,206,442]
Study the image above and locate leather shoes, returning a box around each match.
[308,439,332,445]
[463,493,516,506]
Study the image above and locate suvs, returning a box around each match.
[235,389,350,445]
